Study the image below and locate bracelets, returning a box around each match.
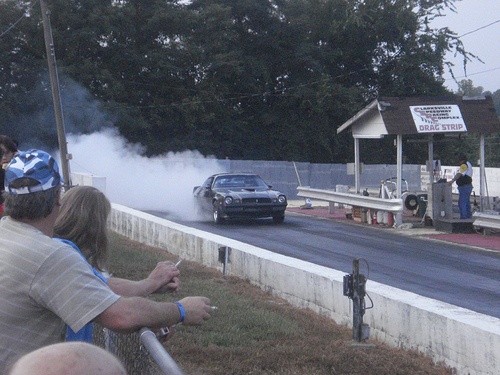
[174,302,185,322]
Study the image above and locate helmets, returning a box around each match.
[244,177,254,186]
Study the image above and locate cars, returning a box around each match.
[193,173,288,225]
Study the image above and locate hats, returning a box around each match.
[3,149,65,194]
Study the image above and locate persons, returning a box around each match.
[0,150,210,375]
[0,135,18,164]
[53,186,180,344]
[448,152,473,219]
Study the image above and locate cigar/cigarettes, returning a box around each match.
[210,307,217,310]
[175,260,183,267]
